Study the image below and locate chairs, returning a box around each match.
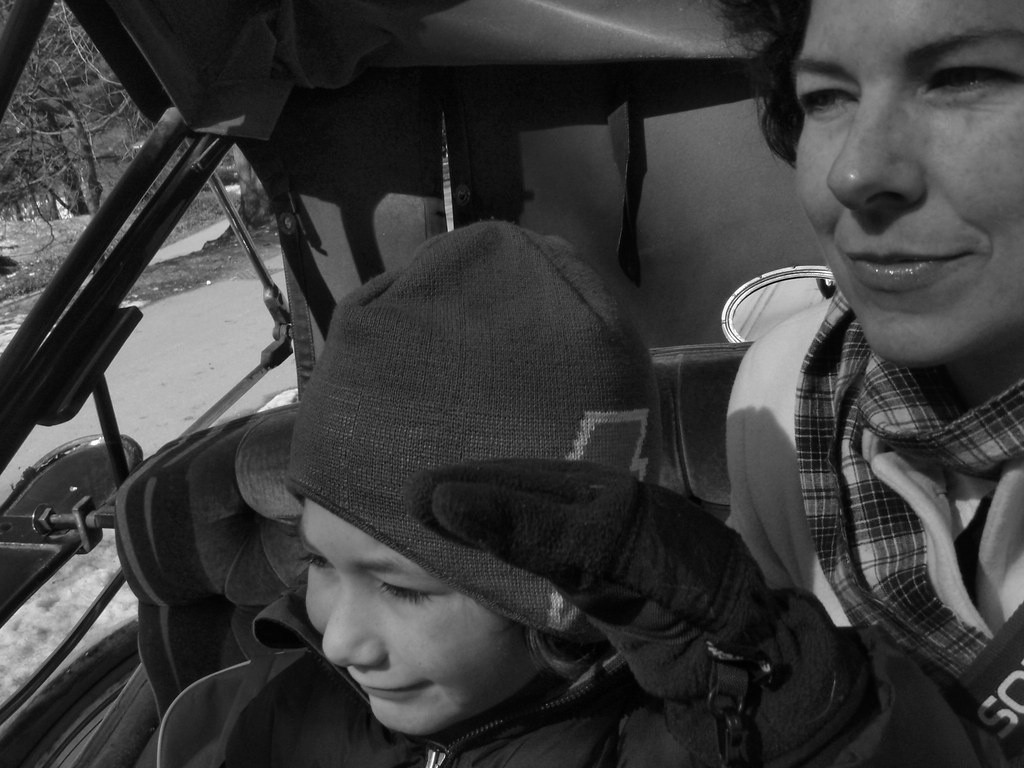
[114,339,754,768]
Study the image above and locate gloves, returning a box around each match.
[404,458,869,768]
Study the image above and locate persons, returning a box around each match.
[127,220,1008,768]
[699,0,1024,768]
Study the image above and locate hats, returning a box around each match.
[289,220,663,640]
